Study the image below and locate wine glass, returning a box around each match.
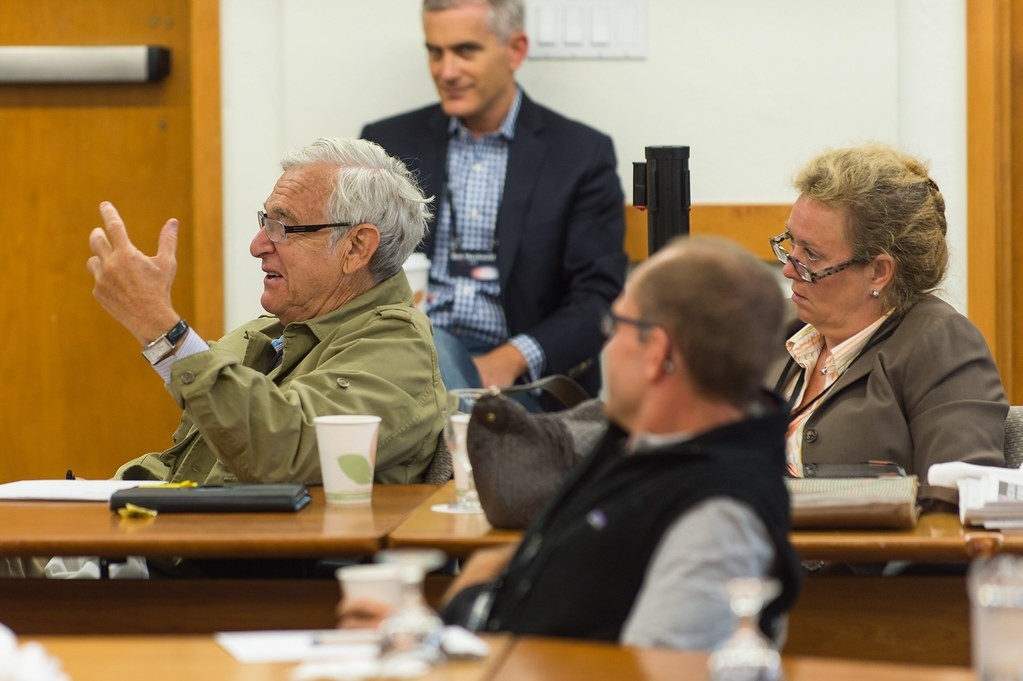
[710,579,782,680]
[441,389,507,504]
[374,548,449,664]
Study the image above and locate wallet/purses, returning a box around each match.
[110,484,310,515]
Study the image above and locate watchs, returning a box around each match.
[141,321,187,365]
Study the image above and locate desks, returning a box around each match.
[0,479,1023,681]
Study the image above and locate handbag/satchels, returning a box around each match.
[466,373,609,531]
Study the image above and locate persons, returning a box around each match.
[0,138,449,581]
[761,144,1009,574]
[357,0,624,412]
[437,236,793,647]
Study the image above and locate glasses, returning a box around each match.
[598,310,675,373]
[769,231,881,284]
[257,210,360,243]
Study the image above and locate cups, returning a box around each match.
[396,250,430,314]
[964,550,1023,681]
[314,414,384,505]
[333,566,406,609]
[448,416,472,494]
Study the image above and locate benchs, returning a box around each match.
[619,144,798,264]
